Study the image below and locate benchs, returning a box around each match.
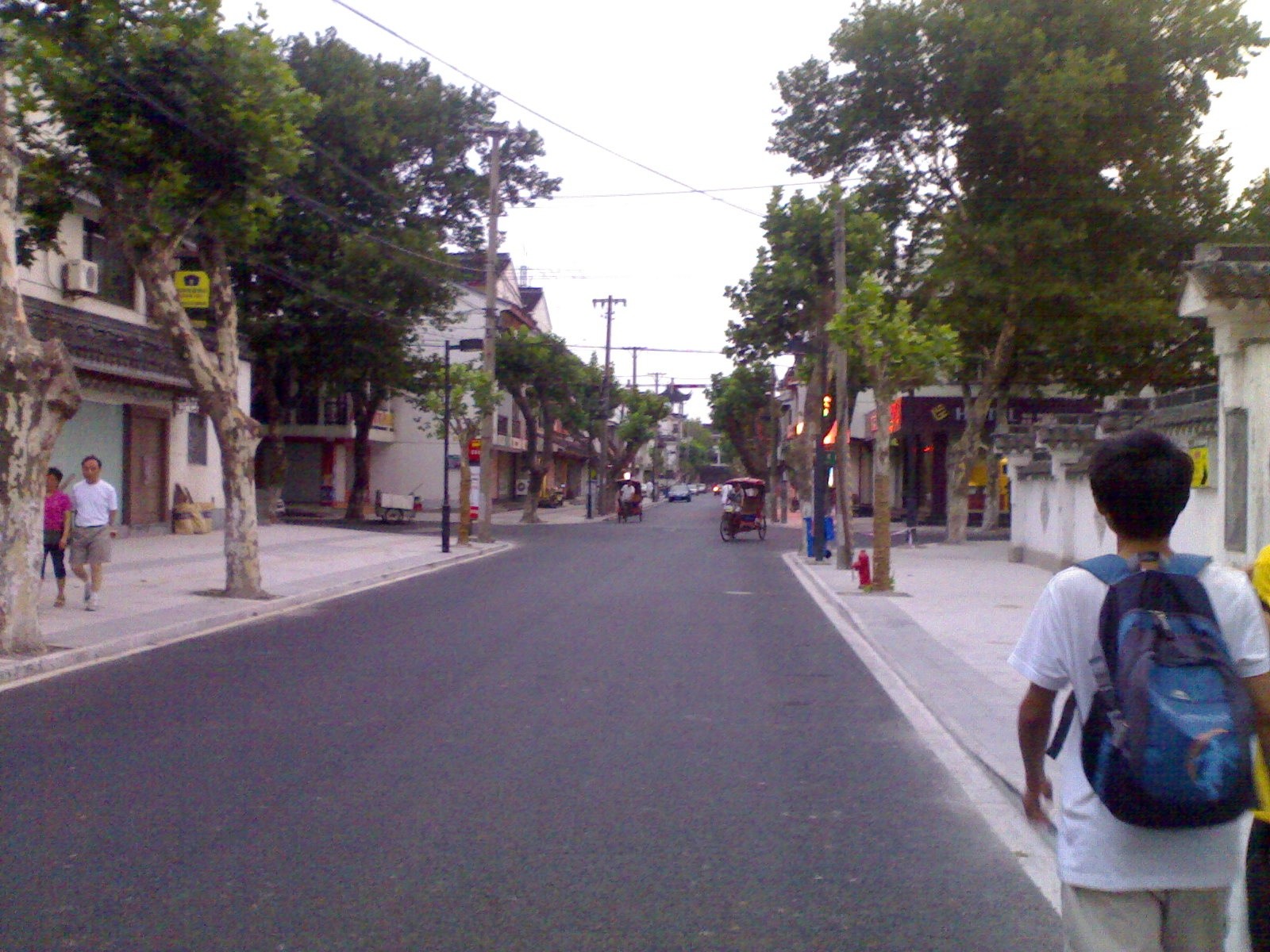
[741,497,765,520]
[632,495,641,506]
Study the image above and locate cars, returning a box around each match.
[687,484,699,496]
[667,484,692,503]
[696,482,723,496]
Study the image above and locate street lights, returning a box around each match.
[441,337,484,552]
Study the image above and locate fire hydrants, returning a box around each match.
[851,548,872,589]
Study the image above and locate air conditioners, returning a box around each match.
[67,259,99,296]
[516,479,528,495]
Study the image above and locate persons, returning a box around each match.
[1006,428,1270,952]
[619,479,635,519]
[70,456,117,612]
[40,467,73,608]
[725,482,744,537]
[1241,546,1270,950]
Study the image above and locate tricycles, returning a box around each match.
[614,478,644,523]
[719,477,768,542]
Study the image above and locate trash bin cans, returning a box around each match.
[805,516,836,557]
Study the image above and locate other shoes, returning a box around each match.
[53,597,65,608]
[86,597,99,610]
[84,581,92,600]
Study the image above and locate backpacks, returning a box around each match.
[1045,553,1265,827]
